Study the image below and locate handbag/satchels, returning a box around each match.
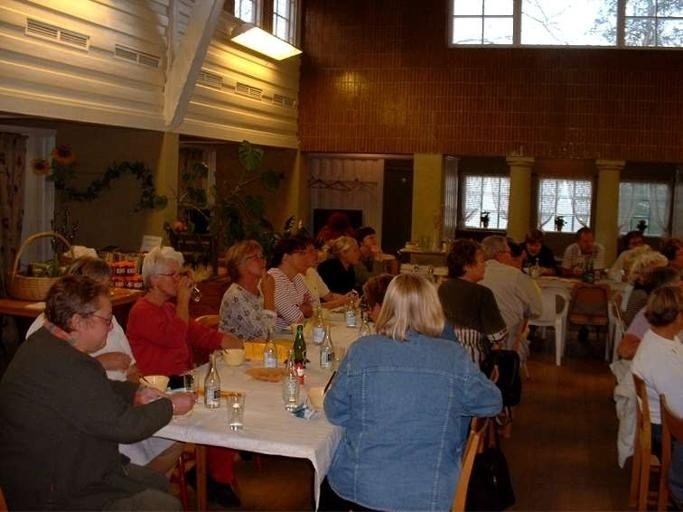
[472,417,516,512]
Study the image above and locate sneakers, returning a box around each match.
[184,466,241,508]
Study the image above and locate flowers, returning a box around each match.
[31,144,167,214]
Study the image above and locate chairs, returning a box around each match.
[195,315,223,335]
[629,371,683,511]
[452,413,490,511]
[522,278,614,368]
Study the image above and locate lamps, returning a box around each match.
[230,24,302,62]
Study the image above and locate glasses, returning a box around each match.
[244,251,267,260]
[88,312,112,326]
[157,271,191,281]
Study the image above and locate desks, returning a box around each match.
[0,288,146,318]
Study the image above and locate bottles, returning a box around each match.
[264,307,334,413]
[441,238,451,253]
[204,354,221,409]
[581,257,596,284]
[344,294,372,338]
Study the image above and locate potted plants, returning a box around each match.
[178,142,284,247]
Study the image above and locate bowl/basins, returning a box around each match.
[222,348,245,367]
[137,375,169,396]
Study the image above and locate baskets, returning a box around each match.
[4,231,76,300]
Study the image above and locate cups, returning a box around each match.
[227,392,246,429]
[530,272,539,278]
[183,371,198,403]
[189,282,204,302]
